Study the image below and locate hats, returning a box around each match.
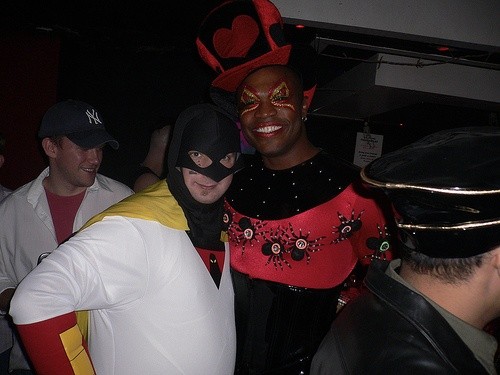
[360,126,500,258]
[38,99,119,151]
[195,0,322,121]
[167,104,246,182]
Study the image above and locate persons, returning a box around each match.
[9,100,246,375]
[0,0,500,375]
[311,126,500,375]
